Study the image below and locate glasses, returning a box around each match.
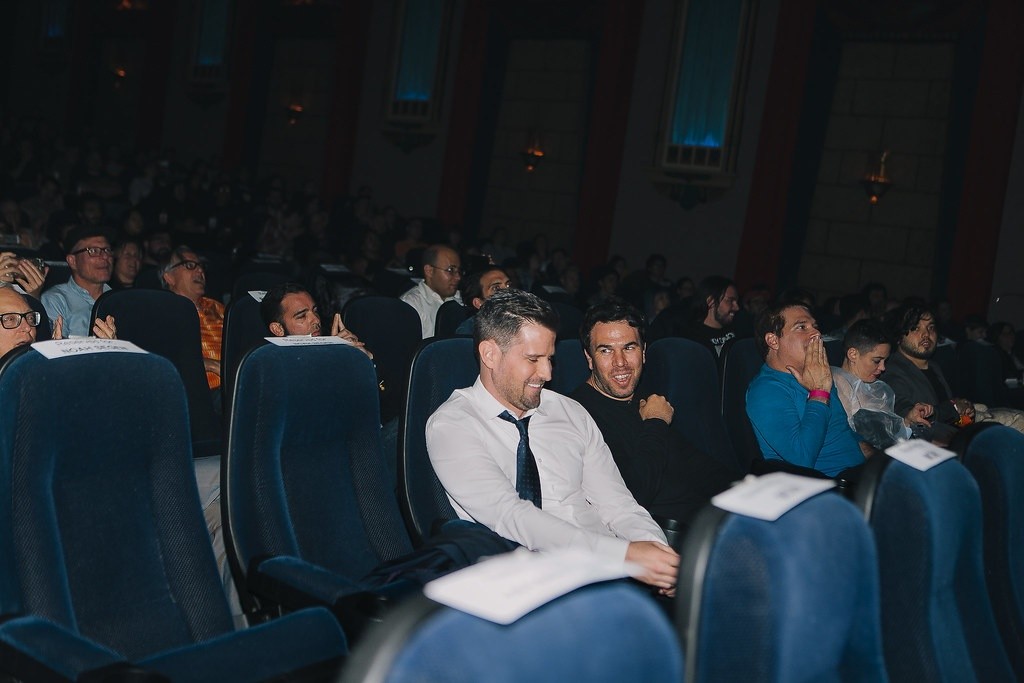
[171,260,209,272]
[430,265,466,276]
[71,246,115,258]
[0,310,41,329]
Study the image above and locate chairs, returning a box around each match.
[0,234,1024,683]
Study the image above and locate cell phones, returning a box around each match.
[5,258,45,279]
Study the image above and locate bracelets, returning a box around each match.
[808,390,830,399]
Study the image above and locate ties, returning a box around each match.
[497,410,542,514]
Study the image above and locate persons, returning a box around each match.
[960,315,1024,379]
[0,109,741,415]
[828,299,874,338]
[745,299,976,477]
[781,290,815,310]
[577,302,742,522]
[425,288,679,597]
